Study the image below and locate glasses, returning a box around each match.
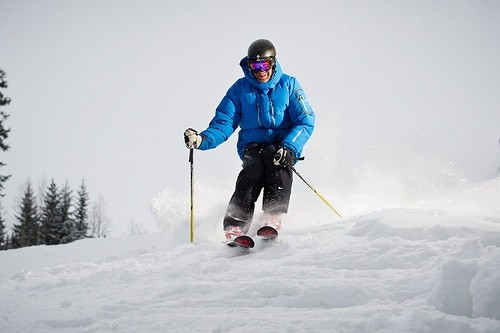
[251,59,274,72]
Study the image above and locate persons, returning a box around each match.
[183,39,315,249]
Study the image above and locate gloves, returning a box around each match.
[273,147,294,170]
[184,128,202,149]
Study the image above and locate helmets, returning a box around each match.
[248,39,277,60]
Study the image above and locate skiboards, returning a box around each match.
[257,226,277,240]
[222,236,254,248]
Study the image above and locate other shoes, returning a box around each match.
[224,226,243,242]
[262,213,282,231]
